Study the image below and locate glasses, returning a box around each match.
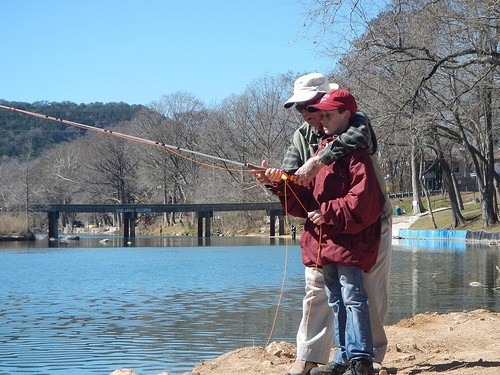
[295,104,311,113]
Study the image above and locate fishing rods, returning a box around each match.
[0,105,298,182]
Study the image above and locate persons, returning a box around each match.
[291,225,296,239]
[247,73,392,375]
[265,89,385,375]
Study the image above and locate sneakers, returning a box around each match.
[311,361,350,375]
[342,359,375,375]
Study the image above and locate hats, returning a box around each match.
[283,73,339,108]
[307,88,358,112]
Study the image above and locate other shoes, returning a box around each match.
[285,360,318,375]
[373,362,382,371]
